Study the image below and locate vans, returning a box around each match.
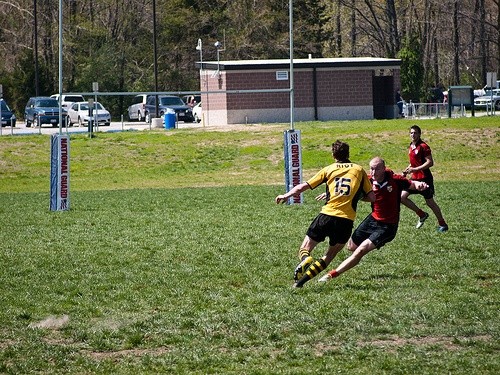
[48,93,86,127]
[181,95,202,109]
[126,93,156,122]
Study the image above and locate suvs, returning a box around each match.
[473,80,500,99]
[24,96,70,128]
[142,95,194,125]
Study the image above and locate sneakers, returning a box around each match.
[433,224,448,232]
[416,212,429,229]
[318,274,332,285]
[292,283,301,289]
[294,255,314,281]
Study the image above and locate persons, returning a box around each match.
[401,125,449,231]
[395,89,405,118]
[314,158,430,283]
[275,140,376,288]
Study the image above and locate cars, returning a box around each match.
[66,101,111,127]
[0,98,17,128]
[191,101,202,124]
[473,88,500,112]
